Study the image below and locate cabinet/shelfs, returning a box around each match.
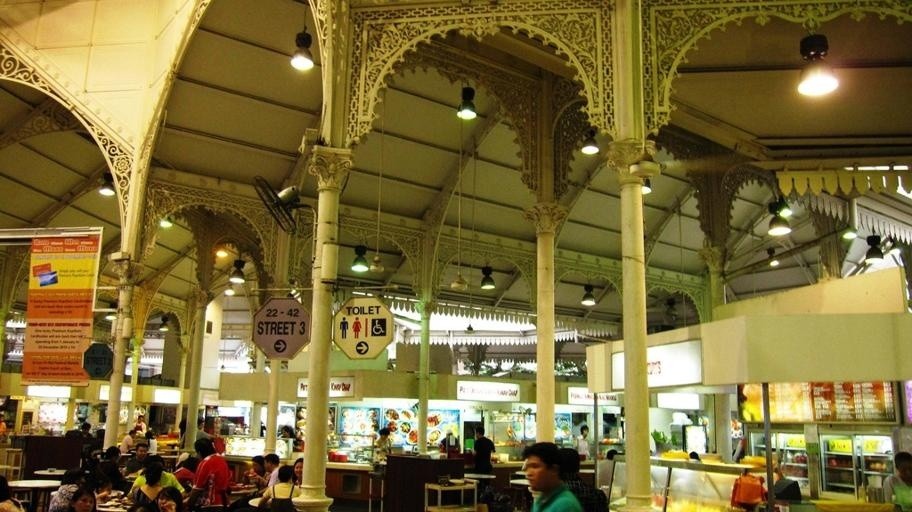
[425,479,479,512]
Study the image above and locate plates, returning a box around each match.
[383,407,445,443]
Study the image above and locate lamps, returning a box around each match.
[98,172,115,196]
[780,197,793,219]
[866,226,884,265]
[290,8,315,72]
[795,32,840,97]
[580,137,600,156]
[642,178,652,196]
[841,223,859,241]
[161,217,171,227]
[582,278,596,307]
[157,316,171,333]
[369,87,386,273]
[768,190,792,237]
[456,86,478,120]
[227,244,246,284]
[350,245,369,273]
[450,117,469,292]
[767,246,778,268]
[479,261,496,290]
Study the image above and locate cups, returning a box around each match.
[325,445,376,464]
[490,451,510,464]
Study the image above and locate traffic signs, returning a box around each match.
[333,295,393,361]
[250,296,311,360]
[84,342,113,378]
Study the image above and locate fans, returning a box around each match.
[255,177,318,266]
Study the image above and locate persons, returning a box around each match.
[607,449,618,459]
[576,425,590,460]
[689,452,700,463]
[470,426,496,499]
[884,452,912,512]
[521,443,584,512]
[377,427,391,453]
[49,411,304,511]
[0,476,25,512]
[558,448,609,512]
[439,430,460,453]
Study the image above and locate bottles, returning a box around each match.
[855,475,885,503]
